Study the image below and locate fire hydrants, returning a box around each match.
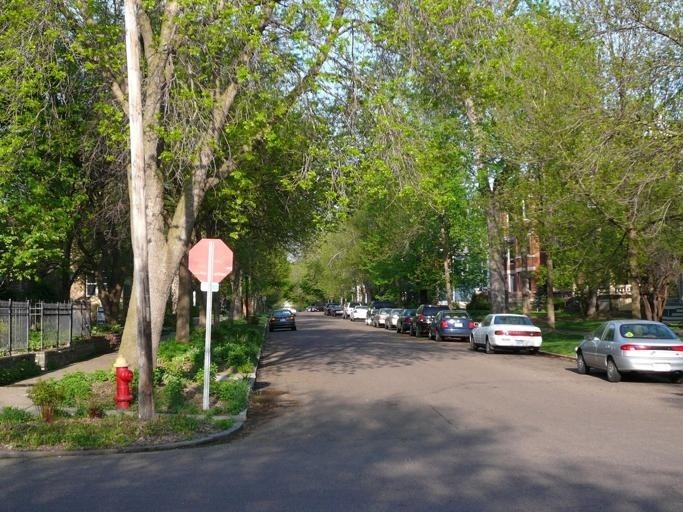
[112,355,134,412]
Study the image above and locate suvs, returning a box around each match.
[410,304,449,337]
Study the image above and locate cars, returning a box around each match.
[575,320,682,381]
[268,310,297,332]
[323,303,369,321]
[367,308,416,334]
[469,313,542,355]
[429,310,475,341]
